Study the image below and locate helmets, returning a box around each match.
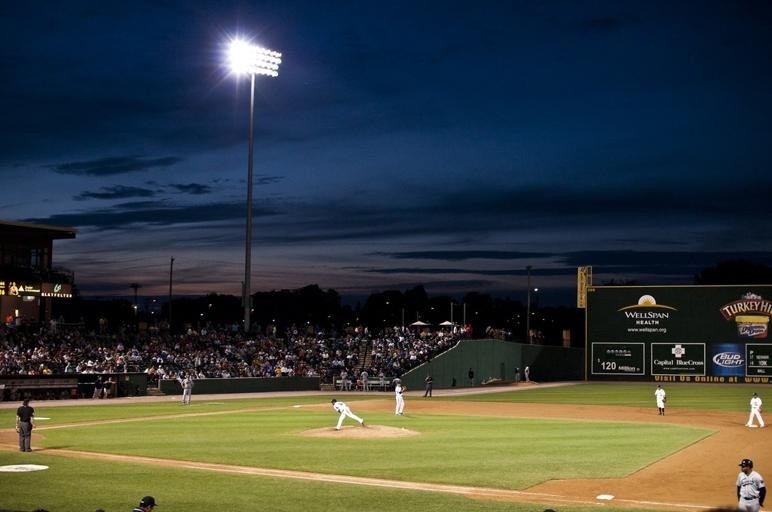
[736,457,753,467]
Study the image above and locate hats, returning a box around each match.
[139,495,159,508]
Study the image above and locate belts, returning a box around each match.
[744,496,757,501]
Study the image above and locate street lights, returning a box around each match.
[221,37,285,336]
[168,256,175,328]
[129,281,143,323]
[526,265,531,335]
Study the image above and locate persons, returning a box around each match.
[330,399,366,431]
[736,458,767,512]
[132,495,159,511]
[392,378,408,416]
[13,399,37,452]
[1,313,474,399]
[654,384,666,416]
[512,366,520,384]
[744,392,765,428]
[180,372,194,406]
[524,366,530,383]
